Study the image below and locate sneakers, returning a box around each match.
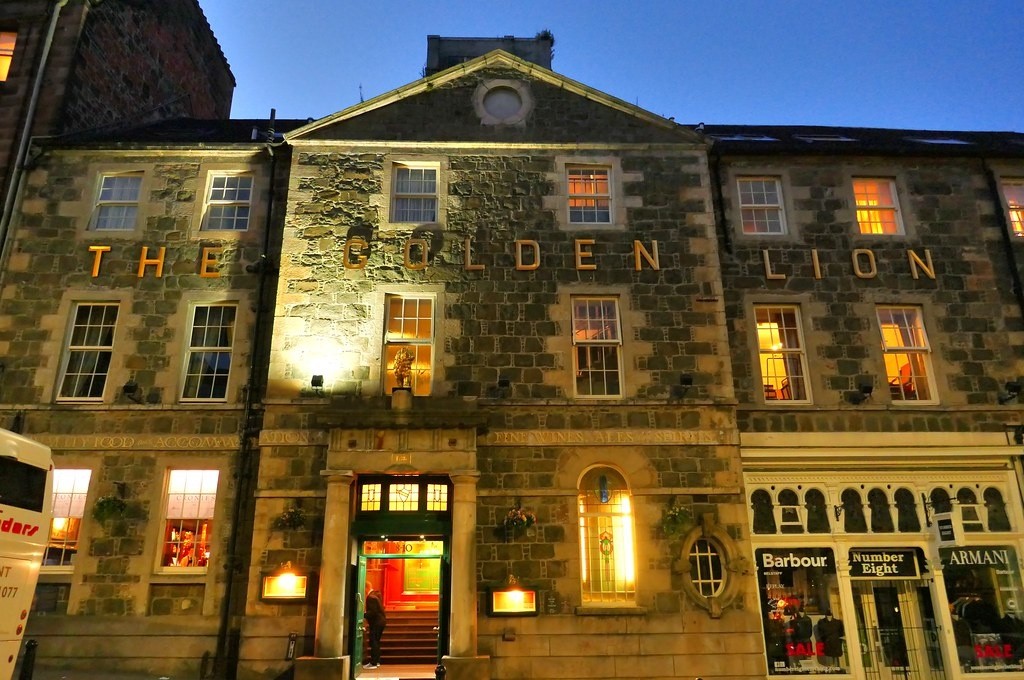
[377,663,380,666]
[363,663,377,670]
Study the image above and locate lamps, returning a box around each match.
[490,580,541,616]
[123,381,143,402]
[261,564,315,602]
[673,373,695,398]
[312,373,323,391]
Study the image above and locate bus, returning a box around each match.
[0,427,55,680]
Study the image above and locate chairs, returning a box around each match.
[888,361,914,400]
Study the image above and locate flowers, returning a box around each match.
[650,505,694,534]
[274,510,307,530]
[504,508,536,525]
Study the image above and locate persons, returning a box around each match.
[362,581,385,669]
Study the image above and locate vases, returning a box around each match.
[506,521,526,538]
